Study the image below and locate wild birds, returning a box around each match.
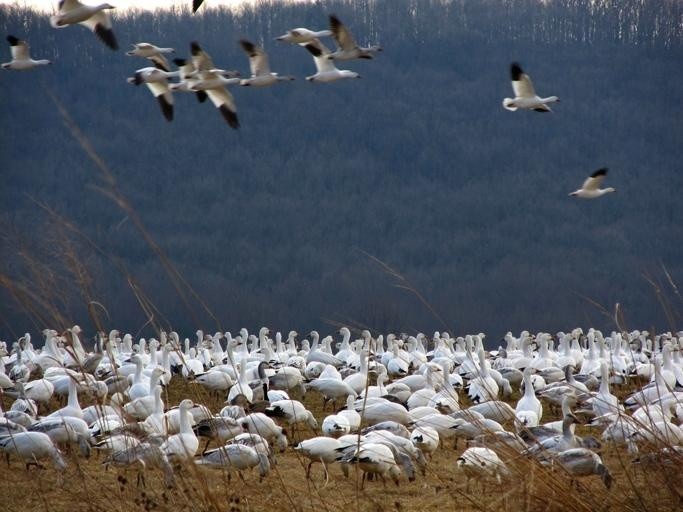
[275,26,336,47]
[125,38,242,132]
[0,324,683,491]
[49,0,121,51]
[326,13,383,61]
[304,42,361,83]
[238,38,296,89]
[502,61,561,114]
[193,0,203,15]
[567,167,617,200]
[0,35,52,71]
